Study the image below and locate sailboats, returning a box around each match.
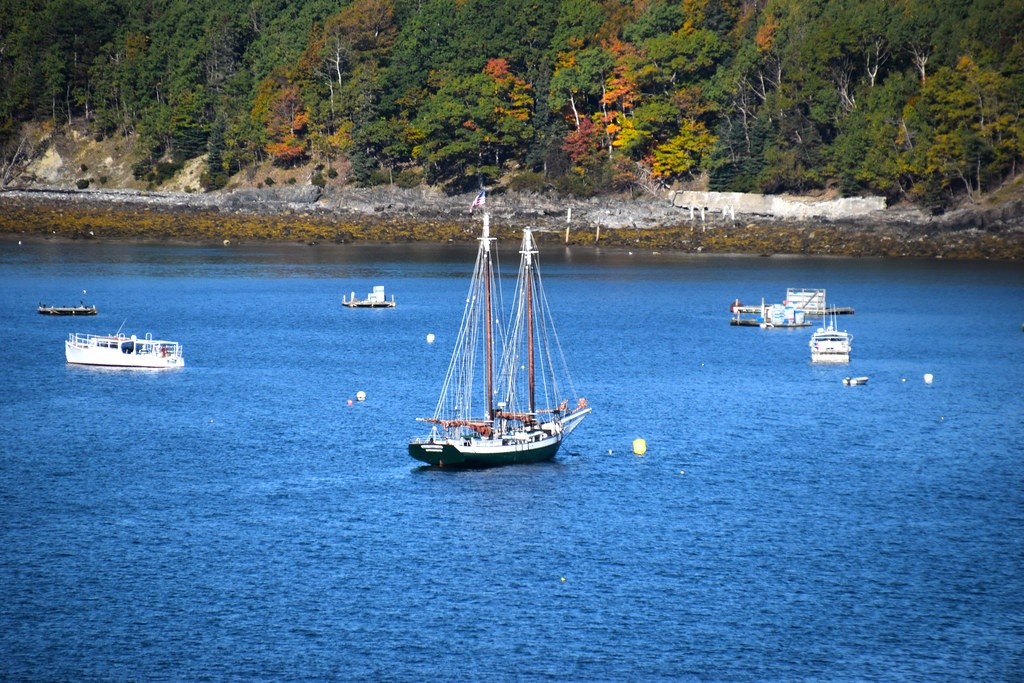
[408,190,591,467]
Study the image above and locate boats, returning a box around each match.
[808,301,854,365]
[64,331,184,367]
[841,377,869,386]
[730,288,853,327]
[341,286,396,308]
[37,303,96,315]
